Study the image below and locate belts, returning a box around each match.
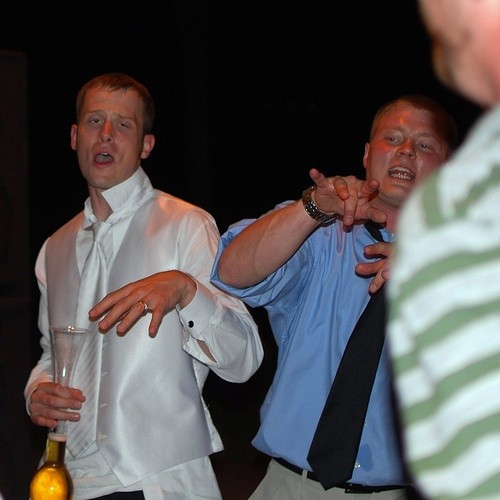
[274,456,404,493]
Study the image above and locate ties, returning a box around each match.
[67,185,147,459]
[306,219,387,492]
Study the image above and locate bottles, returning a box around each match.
[28,432,75,500]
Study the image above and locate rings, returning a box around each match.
[143,300,149,312]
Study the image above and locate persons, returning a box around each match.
[211,93,451,499]
[23,72,264,496]
[383,1,498,499]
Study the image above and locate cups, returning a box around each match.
[49,324,90,436]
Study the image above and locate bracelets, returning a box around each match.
[302,187,338,231]
